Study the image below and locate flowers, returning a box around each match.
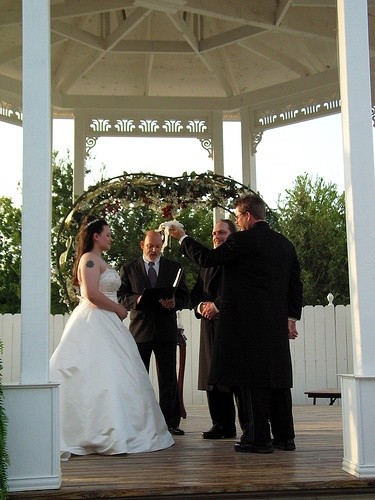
[51,169,284,314]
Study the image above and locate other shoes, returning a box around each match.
[234,441,274,454]
[272,439,296,450]
[169,425,185,435]
[202,424,235,439]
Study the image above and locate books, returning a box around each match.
[134,267,184,312]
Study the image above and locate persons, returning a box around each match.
[170,194,303,455]
[117,230,190,435]
[49,215,175,461]
[190,220,271,443]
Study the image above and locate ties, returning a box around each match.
[148,261,158,287]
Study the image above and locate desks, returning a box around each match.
[304,388,341,405]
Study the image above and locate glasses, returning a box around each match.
[233,212,246,221]
